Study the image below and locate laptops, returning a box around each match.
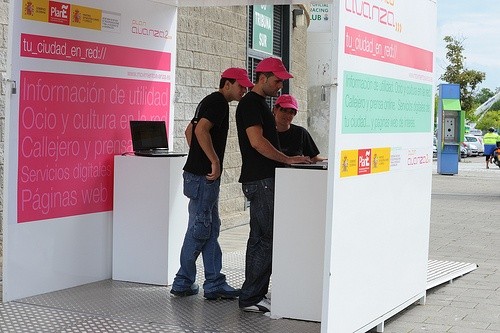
[289,162,329,169]
[130,120,188,157]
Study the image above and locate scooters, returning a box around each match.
[493,147,500,168]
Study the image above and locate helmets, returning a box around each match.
[489,127,494,131]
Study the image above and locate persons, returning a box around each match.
[483,127,500,169]
[170,67,253,299]
[236,56,313,316]
[273,95,322,166]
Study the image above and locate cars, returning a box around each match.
[433,129,484,162]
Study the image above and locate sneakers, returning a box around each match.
[240,297,271,314]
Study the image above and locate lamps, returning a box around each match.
[293,8,305,29]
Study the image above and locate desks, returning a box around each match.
[269,168,328,322]
[113,154,191,287]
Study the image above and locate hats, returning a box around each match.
[255,57,294,80]
[275,94,298,111]
[221,67,254,88]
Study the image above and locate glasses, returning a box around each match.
[277,106,295,115]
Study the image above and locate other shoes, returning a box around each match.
[203,283,241,298]
[490,158,492,164]
[169,285,199,297]
[486,167,489,169]
